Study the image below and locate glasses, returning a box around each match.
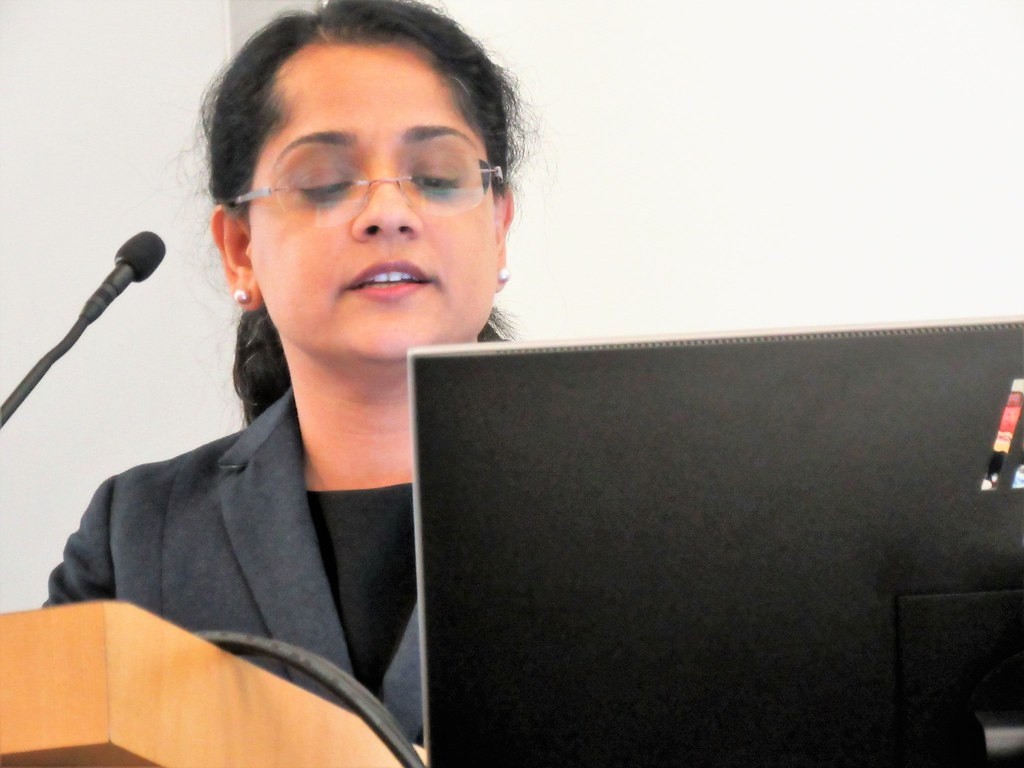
[231,149,503,230]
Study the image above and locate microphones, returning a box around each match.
[0,232,166,429]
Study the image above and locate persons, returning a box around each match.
[40,0,524,768]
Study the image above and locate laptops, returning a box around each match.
[408,313,1024,768]
